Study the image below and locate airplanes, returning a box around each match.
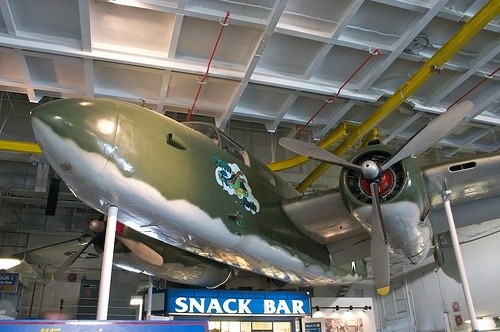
[11,77,499,297]
